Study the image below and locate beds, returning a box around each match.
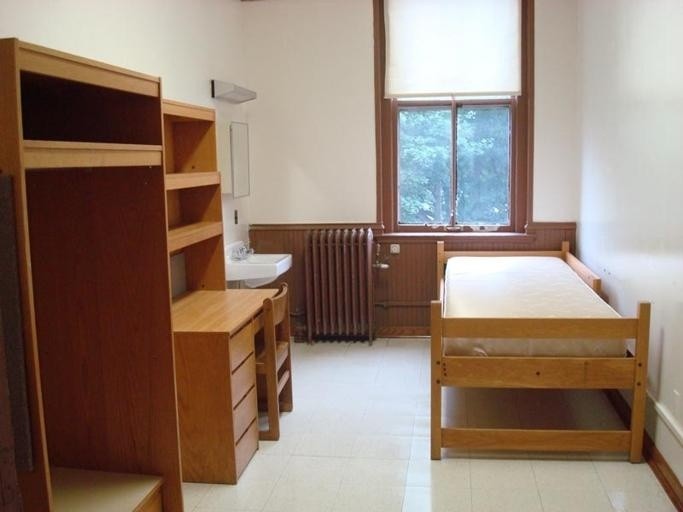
[429,240,650,463]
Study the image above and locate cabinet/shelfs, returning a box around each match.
[162,99,280,486]
[1,36,184,511]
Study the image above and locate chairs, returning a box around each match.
[254,281,293,440]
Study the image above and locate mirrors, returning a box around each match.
[230,120,250,198]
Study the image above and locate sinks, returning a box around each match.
[225,240,293,288]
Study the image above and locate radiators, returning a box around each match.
[300,227,375,344]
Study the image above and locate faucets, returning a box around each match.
[245,248,255,256]
